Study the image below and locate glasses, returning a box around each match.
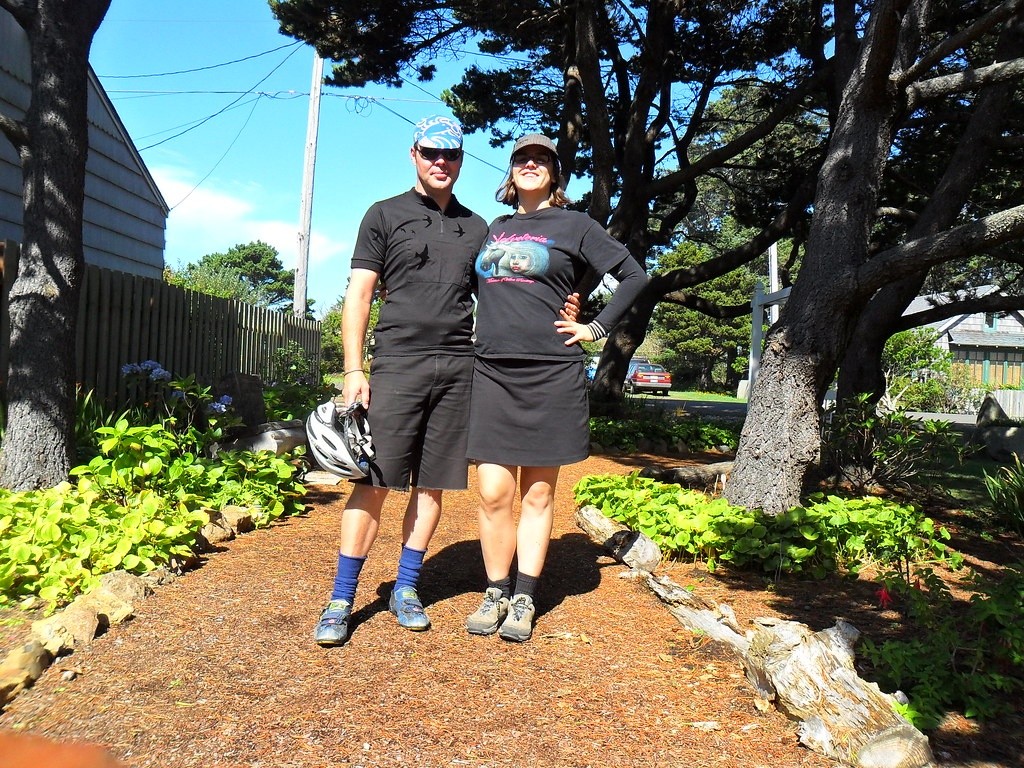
[414,147,461,161]
[513,153,552,164]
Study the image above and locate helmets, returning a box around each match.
[305,400,370,479]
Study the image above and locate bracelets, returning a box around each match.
[343,369,365,377]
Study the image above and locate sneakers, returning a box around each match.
[389,585,429,630]
[467,588,509,637]
[314,599,351,647]
[497,594,535,642]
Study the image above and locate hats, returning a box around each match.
[412,115,463,150]
[511,132,558,158]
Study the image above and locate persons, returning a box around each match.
[464,133,650,643]
[313,115,582,645]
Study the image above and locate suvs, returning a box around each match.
[627,356,652,376]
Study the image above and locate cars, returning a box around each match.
[584,356,600,380]
[624,363,673,395]
